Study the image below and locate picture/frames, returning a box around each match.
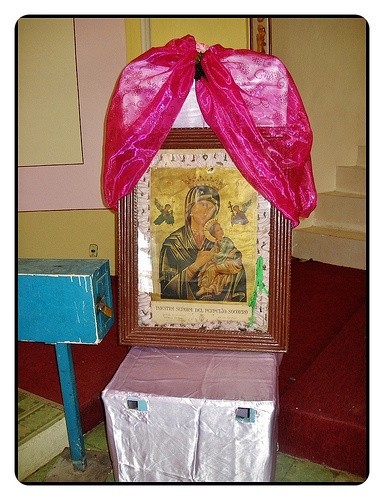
[115,126,293,353]
